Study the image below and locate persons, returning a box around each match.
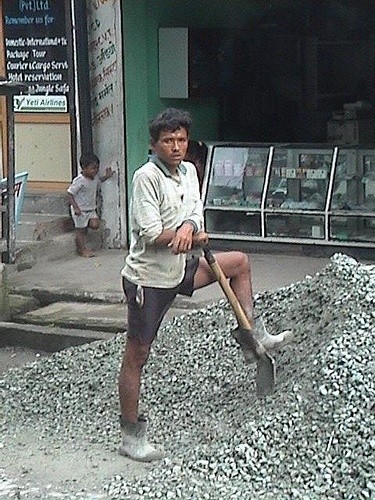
[66,152,116,257]
[117,109,295,463]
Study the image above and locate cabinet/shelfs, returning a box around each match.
[194,141,375,253]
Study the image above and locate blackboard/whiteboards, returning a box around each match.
[0,0,70,114]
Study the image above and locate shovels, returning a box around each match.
[201,240,275,396]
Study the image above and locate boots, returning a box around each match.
[117,416,164,460]
[231,316,294,364]
